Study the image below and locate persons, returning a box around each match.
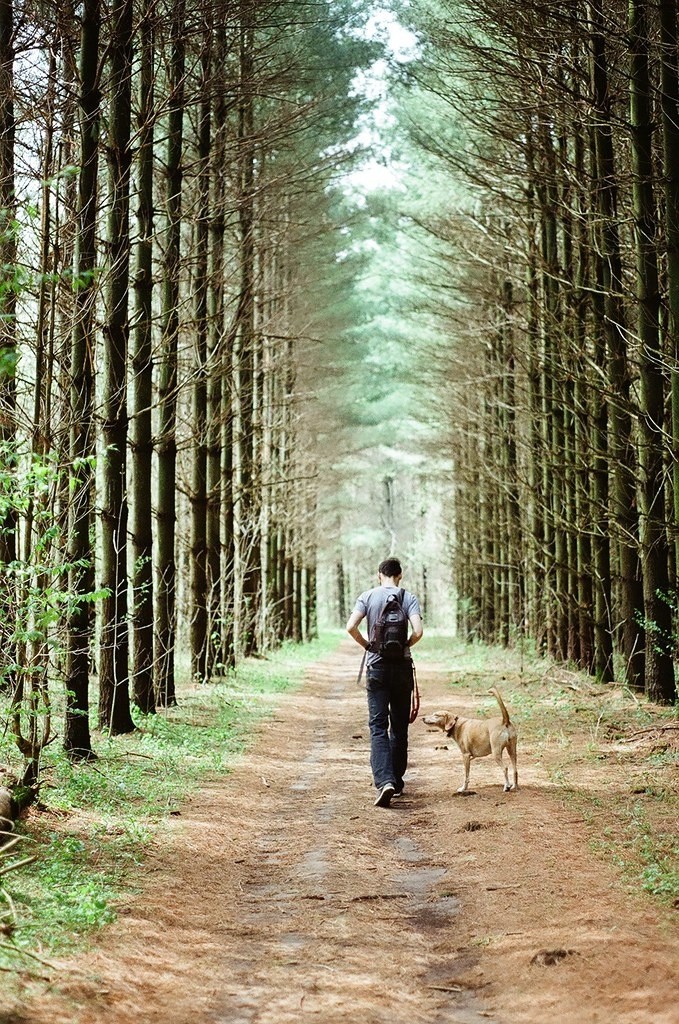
[347,559,423,806]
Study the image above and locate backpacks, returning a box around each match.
[373,588,407,658]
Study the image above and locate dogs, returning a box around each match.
[422,688,519,792]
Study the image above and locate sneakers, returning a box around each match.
[393,790,402,797]
[374,783,395,807]
